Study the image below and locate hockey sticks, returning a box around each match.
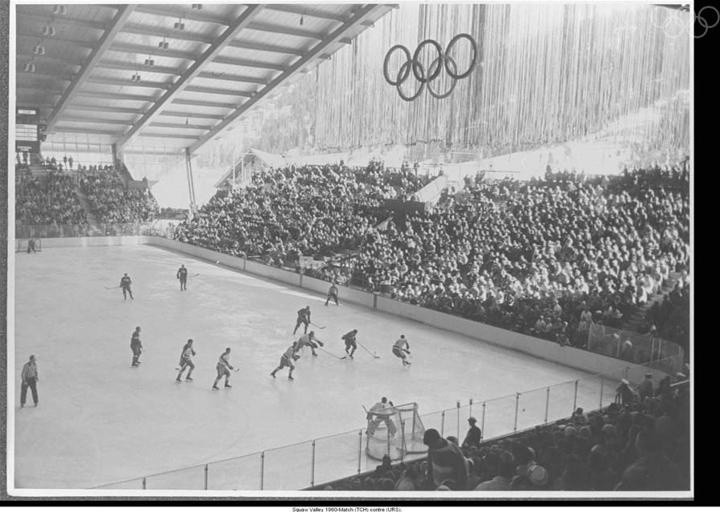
[188,273,198,277]
[308,321,328,330]
[361,405,386,431]
[175,354,195,373]
[356,340,382,361]
[104,285,127,290]
[317,347,348,360]
[233,367,240,373]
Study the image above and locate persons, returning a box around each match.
[15,147,318,278]
[209,345,236,389]
[177,265,187,291]
[177,338,197,383]
[129,326,143,368]
[271,341,300,380]
[296,330,323,357]
[318,150,690,489]
[294,306,312,335]
[119,273,134,300]
[19,354,40,406]
